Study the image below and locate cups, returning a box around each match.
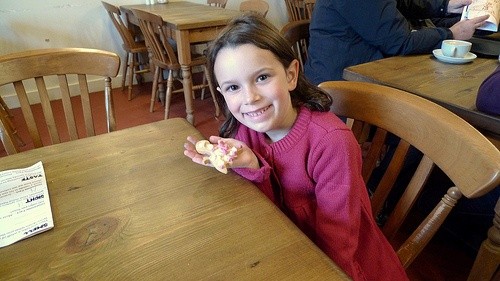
[441,39,472,58]
[145,0,167,5]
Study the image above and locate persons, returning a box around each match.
[183,12,408,281]
[304,0,500,281]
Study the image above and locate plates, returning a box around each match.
[433,48,477,65]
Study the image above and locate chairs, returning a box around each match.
[0,48,121,155]
[100,0,316,119]
[317,81,500,267]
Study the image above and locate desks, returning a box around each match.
[120,2,244,125]
[0,117,354,281]
[344,54,500,281]
